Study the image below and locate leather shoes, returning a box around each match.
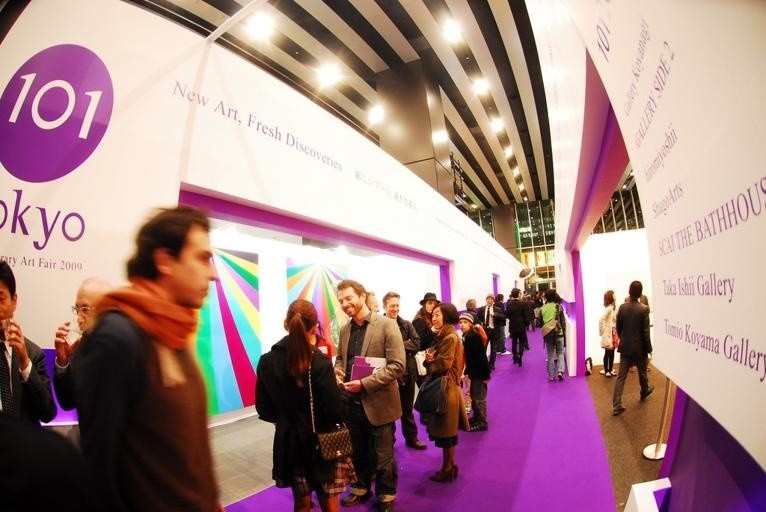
[372,500,395,512]
[340,489,374,507]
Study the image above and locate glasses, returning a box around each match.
[71,305,95,318]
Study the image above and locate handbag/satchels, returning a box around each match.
[541,304,562,353]
[307,350,354,462]
[414,332,459,416]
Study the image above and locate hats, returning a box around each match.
[419,292,441,306]
[459,312,474,324]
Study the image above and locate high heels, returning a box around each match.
[435,464,459,478]
[430,467,456,482]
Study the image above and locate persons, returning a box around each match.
[522,291,527,301]
[458,313,491,432]
[414,302,471,483]
[311,335,333,361]
[62,207,219,512]
[478,295,505,370]
[383,292,427,449]
[612,281,654,415]
[536,289,566,381]
[366,293,380,315]
[412,293,441,426]
[0,260,58,512]
[519,293,530,350]
[466,299,481,326]
[598,290,620,376]
[53,276,114,411]
[625,294,651,372]
[506,288,527,367]
[537,293,543,328]
[334,279,406,512]
[524,295,535,332]
[506,295,512,339]
[254,299,358,512]
[495,294,511,355]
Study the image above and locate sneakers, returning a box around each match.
[640,384,654,400]
[606,371,612,377]
[610,369,617,375]
[405,439,427,450]
[557,372,564,381]
[470,421,487,431]
[549,376,555,380]
[613,405,626,415]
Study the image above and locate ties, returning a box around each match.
[0,328,16,417]
[485,307,490,327]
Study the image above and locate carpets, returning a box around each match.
[219,327,619,512]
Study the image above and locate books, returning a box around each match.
[350,356,386,380]
